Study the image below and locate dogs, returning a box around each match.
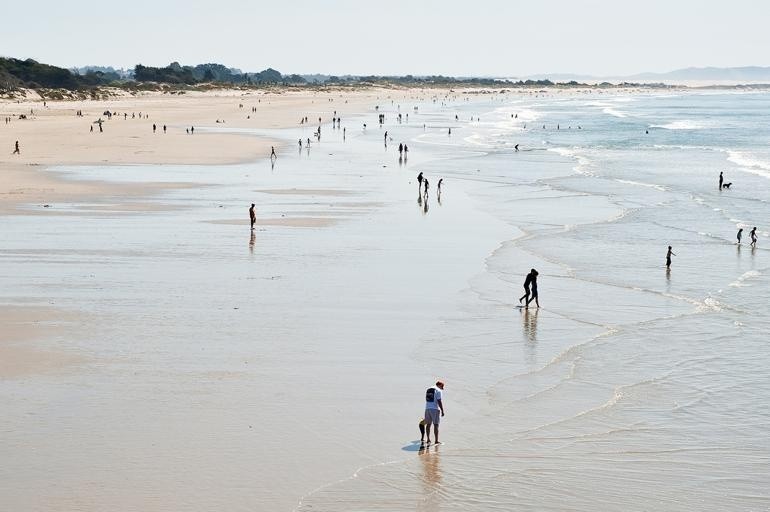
[723,182,732,188]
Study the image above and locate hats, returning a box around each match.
[437,381,444,390]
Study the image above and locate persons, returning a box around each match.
[427,444,443,483]
[719,171,724,190]
[418,415,426,441]
[736,228,744,244]
[522,307,528,338]
[528,271,540,307]
[665,245,676,268]
[163,124,167,134]
[514,144,519,152]
[248,203,256,229]
[748,227,761,247]
[423,197,429,213]
[423,178,430,197]
[436,189,442,206]
[417,187,422,207]
[518,268,536,306]
[152,122,157,133]
[98,121,103,131]
[436,178,444,193]
[424,380,445,444]
[268,110,409,165]
[417,172,425,188]
[248,229,258,250]
[529,308,540,339]
[12,140,20,153]
[417,441,428,464]
